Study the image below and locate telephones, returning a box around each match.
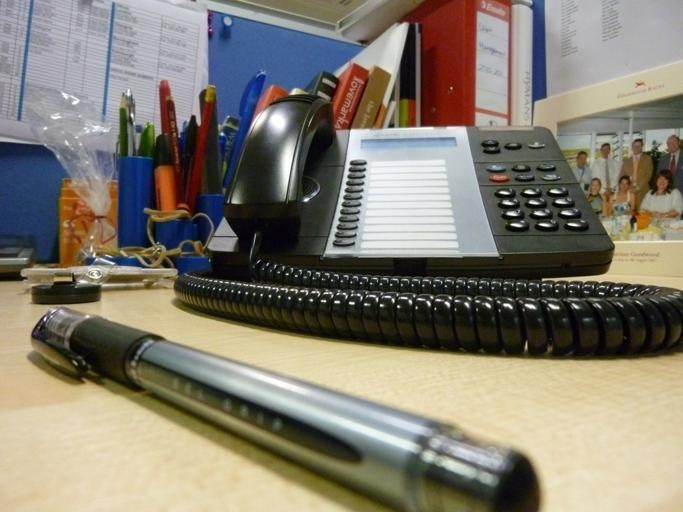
[207,94,616,275]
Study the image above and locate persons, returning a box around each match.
[571,134,683,231]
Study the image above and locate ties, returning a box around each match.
[605,160,610,187]
[670,156,676,177]
[634,159,638,177]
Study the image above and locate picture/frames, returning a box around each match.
[531,58,683,281]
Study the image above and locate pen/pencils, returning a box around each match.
[120,67,268,211]
[30,307,541,511]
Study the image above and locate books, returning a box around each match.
[223,23,415,188]
